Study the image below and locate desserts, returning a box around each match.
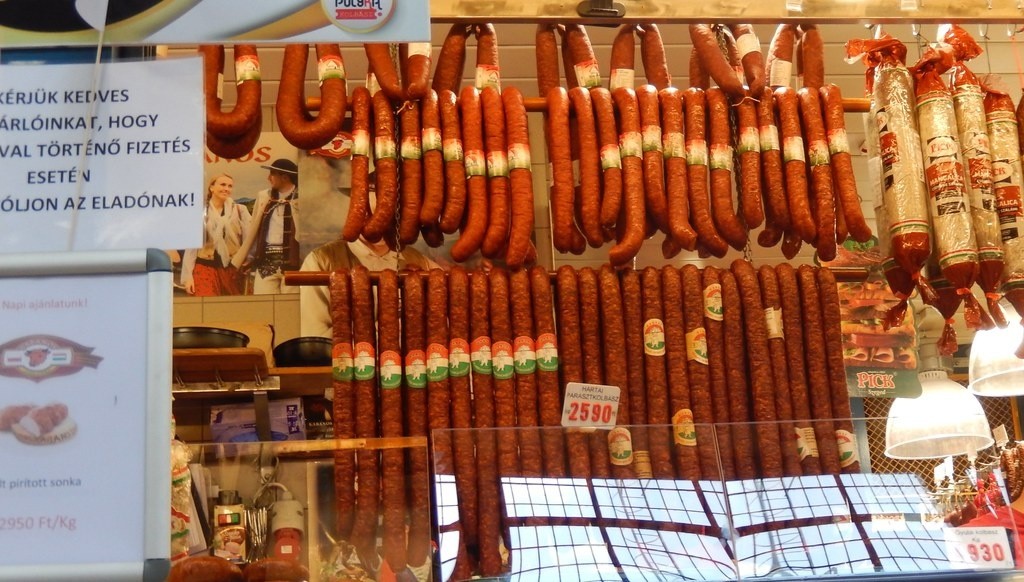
[12,404,76,445]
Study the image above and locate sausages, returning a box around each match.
[169,18,1024,582]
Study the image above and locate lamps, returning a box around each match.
[883,303,996,460]
[966,302,1024,397]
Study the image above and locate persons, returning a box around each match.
[300,170,445,339]
[180,158,299,297]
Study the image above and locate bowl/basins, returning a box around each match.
[172,326,251,348]
[273,336,334,367]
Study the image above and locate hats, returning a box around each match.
[261,159,298,174]
